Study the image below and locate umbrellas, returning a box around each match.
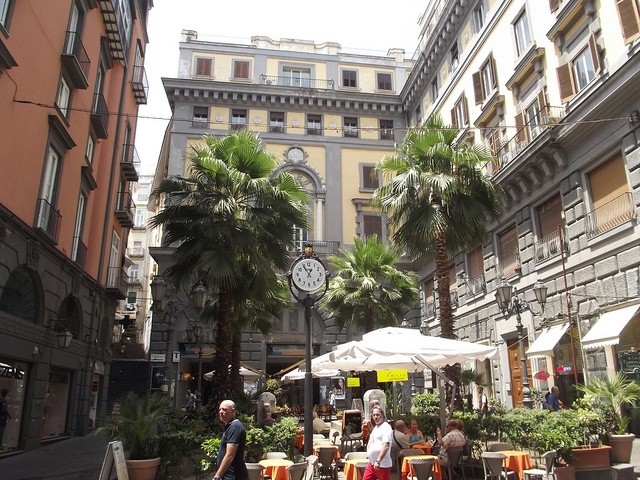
[280,325,500,432]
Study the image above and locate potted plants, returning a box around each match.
[91,387,203,479]
[546,429,576,480]
[572,370,640,463]
[571,409,612,470]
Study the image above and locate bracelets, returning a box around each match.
[213,475,221,480]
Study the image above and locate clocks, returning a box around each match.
[292,259,326,292]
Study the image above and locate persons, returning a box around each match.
[542,392,549,410]
[182,389,200,406]
[211,399,249,480]
[405,420,424,448]
[547,385,560,413]
[361,405,393,480]
[391,418,409,459]
[475,384,489,418]
[327,388,337,413]
[434,419,463,467]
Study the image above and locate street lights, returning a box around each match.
[494,278,548,409]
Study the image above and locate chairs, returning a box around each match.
[261,451,288,460]
[480,453,508,480]
[487,443,512,451]
[244,462,266,480]
[390,447,400,472]
[355,461,368,480]
[312,447,340,480]
[523,449,557,480]
[285,461,308,480]
[344,452,368,461]
[395,448,425,480]
[458,439,475,480]
[302,454,319,480]
[439,446,466,479]
[407,457,436,480]
